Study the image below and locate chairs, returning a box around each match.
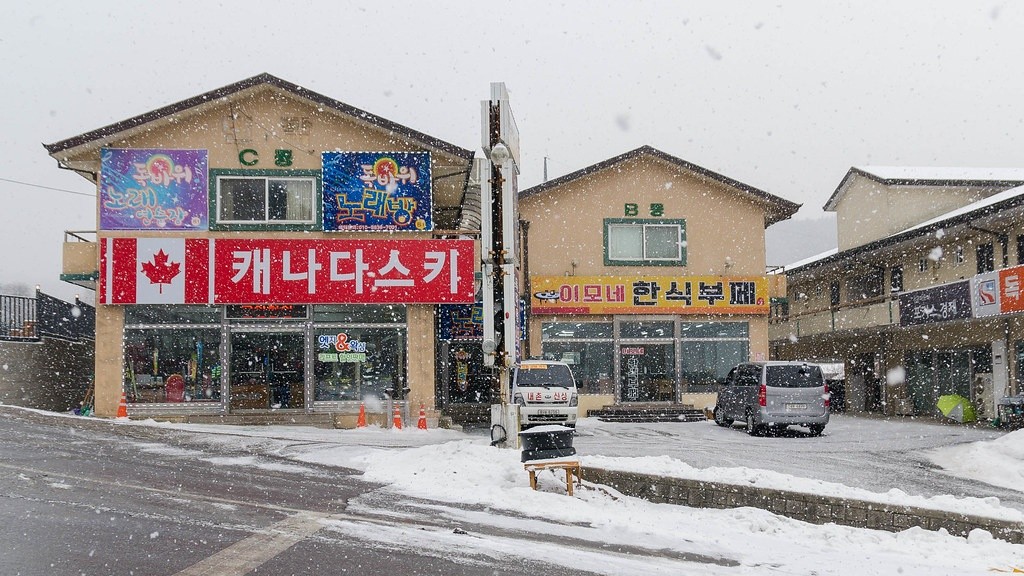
[657,378,674,401]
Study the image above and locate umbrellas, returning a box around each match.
[937,395,976,423]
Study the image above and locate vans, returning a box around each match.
[510,359,583,429]
[713,361,831,436]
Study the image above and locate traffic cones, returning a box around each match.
[393,404,402,429]
[417,404,427,430]
[356,403,366,428]
[116,392,127,419]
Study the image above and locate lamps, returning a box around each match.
[725,256,732,269]
